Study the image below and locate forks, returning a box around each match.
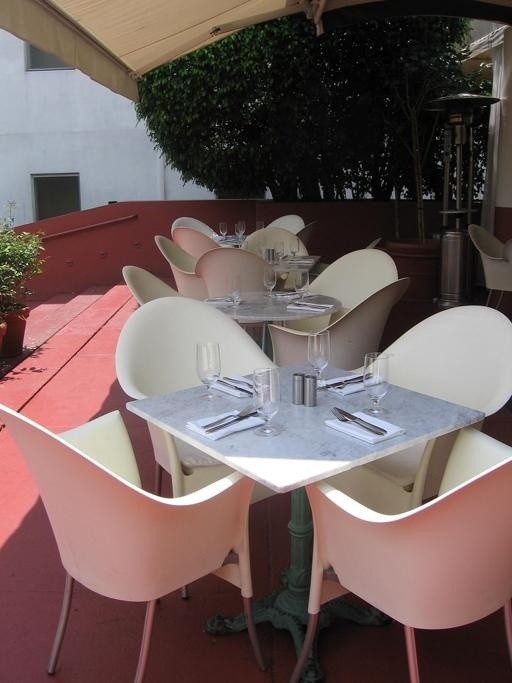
[202,373,388,436]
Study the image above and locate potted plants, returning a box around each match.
[0,196,51,359]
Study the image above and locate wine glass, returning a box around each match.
[254,368,282,436]
[292,269,310,302]
[273,241,285,263]
[226,272,243,310]
[263,265,278,297]
[195,341,221,400]
[288,236,300,262]
[308,330,332,385]
[219,220,246,242]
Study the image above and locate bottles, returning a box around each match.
[265,248,274,262]
